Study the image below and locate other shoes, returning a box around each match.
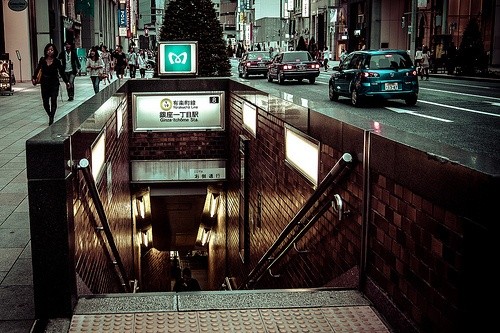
[68,96,73,101]
[49,116,53,125]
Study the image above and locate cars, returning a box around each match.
[237,50,274,79]
[328,49,420,108]
[265,51,321,85]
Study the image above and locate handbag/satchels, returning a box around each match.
[98,73,102,81]
[36,69,41,83]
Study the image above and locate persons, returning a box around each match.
[228,35,366,72]
[31,41,158,126]
[169,256,202,292]
[0,64,9,78]
[416,46,456,81]
